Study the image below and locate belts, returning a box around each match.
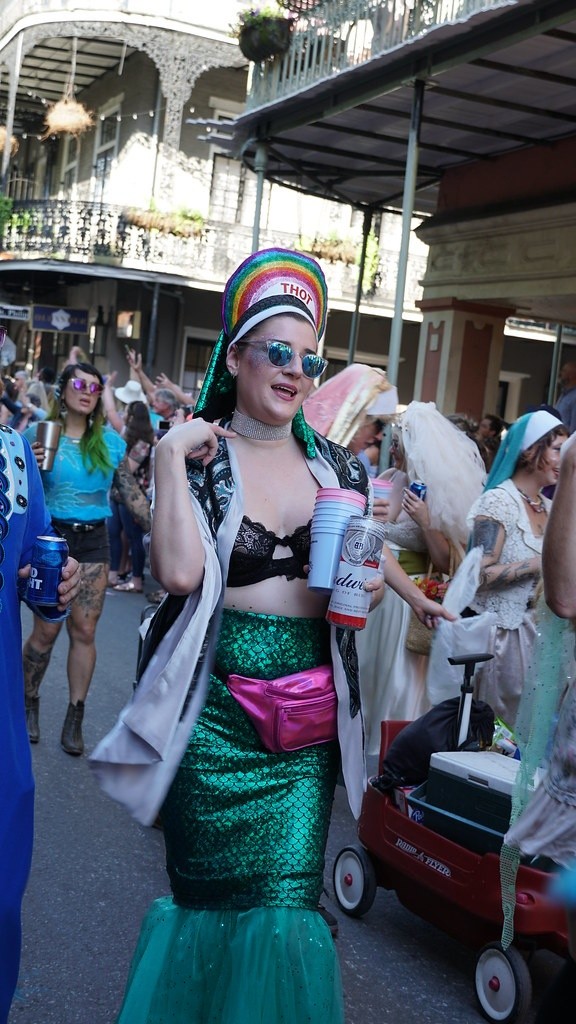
[51,519,105,532]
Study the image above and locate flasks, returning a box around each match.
[35,421,62,473]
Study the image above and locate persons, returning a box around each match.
[299,360,576,951]
[87,247,388,1024]
[0,326,197,754]
[0,423,82,1024]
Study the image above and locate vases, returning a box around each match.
[239,19,292,62]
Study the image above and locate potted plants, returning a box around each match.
[293,227,387,300]
[120,201,204,241]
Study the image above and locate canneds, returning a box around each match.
[489,737,517,757]
[326,516,386,633]
[26,535,70,607]
[409,479,428,502]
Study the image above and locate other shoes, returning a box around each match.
[147,588,167,603]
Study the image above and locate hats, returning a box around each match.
[114,380,148,405]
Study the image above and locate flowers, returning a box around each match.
[228,7,294,49]
[414,578,448,601]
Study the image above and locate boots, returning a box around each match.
[61,700,84,755]
[24,694,41,743]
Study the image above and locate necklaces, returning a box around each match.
[230,407,293,441]
[519,492,542,505]
[516,487,545,513]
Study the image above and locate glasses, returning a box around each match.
[367,416,387,434]
[235,339,330,379]
[67,377,105,395]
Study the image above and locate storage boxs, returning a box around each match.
[405,750,555,873]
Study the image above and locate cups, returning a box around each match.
[306,487,368,596]
[370,477,394,500]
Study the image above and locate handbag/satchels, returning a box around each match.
[213,662,339,753]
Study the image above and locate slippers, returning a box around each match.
[115,581,144,593]
